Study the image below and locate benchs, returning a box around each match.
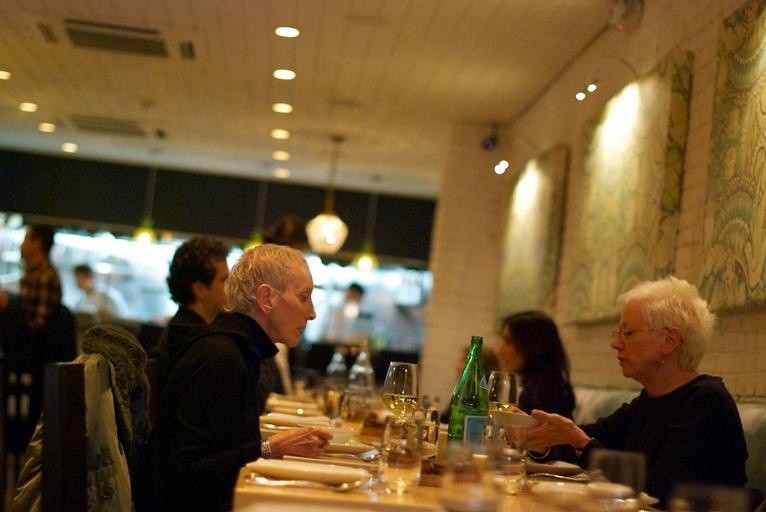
[577,384,766,509]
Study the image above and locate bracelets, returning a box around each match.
[260,438,272,458]
[528,446,552,460]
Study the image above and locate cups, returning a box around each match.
[376,425,749,512]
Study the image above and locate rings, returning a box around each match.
[319,439,327,448]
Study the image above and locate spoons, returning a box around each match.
[316,448,381,460]
[251,475,370,491]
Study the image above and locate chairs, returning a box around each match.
[42,355,131,512]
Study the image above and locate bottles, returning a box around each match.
[445,333,491,445]
[321,344,374,419]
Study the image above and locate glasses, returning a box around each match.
[613,327,665,339]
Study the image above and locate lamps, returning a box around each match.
[303,137,348,256]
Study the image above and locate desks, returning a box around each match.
[229,361,653,512]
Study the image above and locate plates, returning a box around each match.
[246,460,368,483]
[493,411,538,426]
[322,438,372,454]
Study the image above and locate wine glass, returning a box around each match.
[487,370,517,428]
[382,361,417,440]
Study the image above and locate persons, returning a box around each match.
[498,311,576,453]
[455,342,501,409]
[151,236,230,390]
[0,223,62,365]
[324,282,363,341]
[75,265,127,323]
[130,240,335,511]
[502,279,749,511]
